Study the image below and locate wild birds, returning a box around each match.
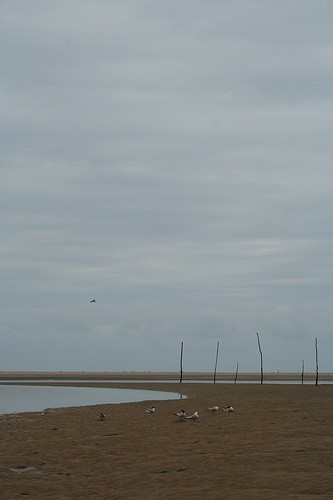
[173,409,199,422]
[90,299,96,303]
[145,406,156,414]
[96,412,107,420]
[206,405,235,414]
